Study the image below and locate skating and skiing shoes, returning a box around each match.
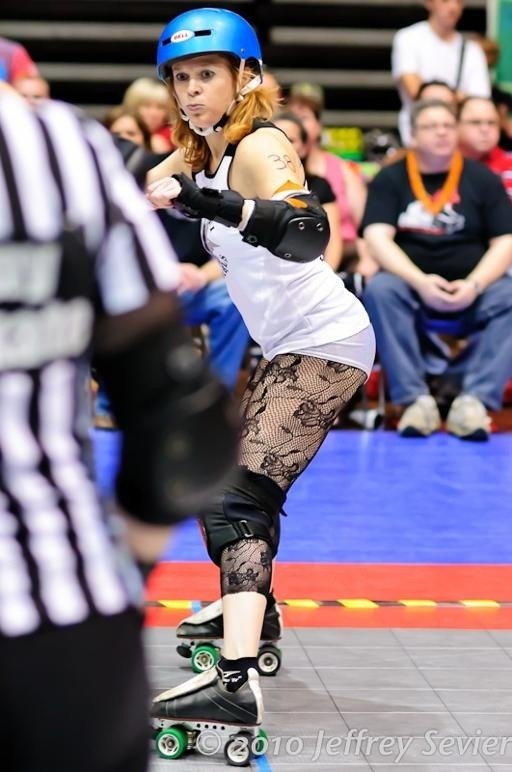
[149,660,268,767]
[176,589,283,678]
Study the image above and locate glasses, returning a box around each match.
[413,122,457,133]
[461,118,498,130]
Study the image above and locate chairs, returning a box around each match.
[354,269,512,432]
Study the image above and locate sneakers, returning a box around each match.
[397,395,444,438]
[446,394,492,444]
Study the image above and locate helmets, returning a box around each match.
[154,5,263,82]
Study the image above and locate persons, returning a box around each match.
[3,78,246,772]
[140,8,380,733]
[0,1,512,443]
